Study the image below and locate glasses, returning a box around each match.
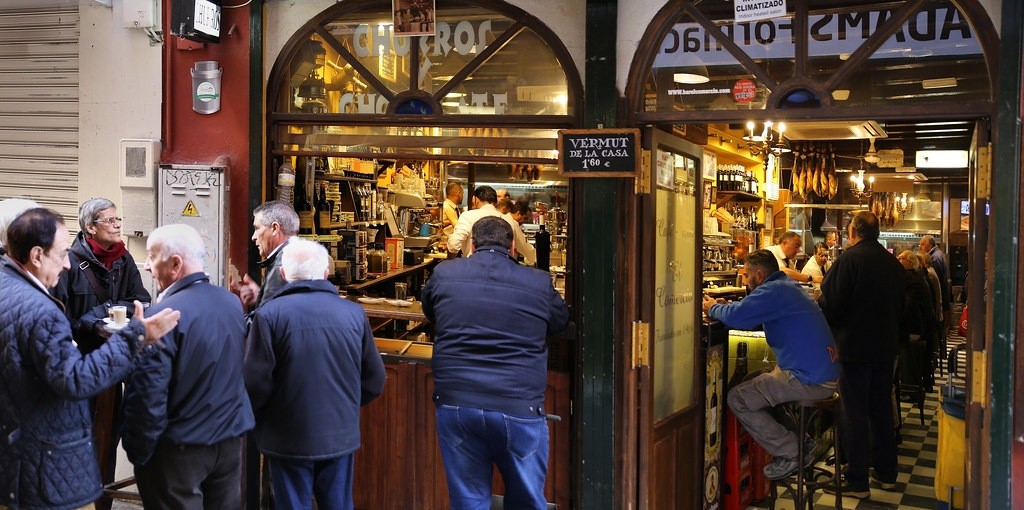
[93,218,122,224]
[918,243,930,247]
[898,257,912,263]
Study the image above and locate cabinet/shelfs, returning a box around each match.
[312,173,440,340]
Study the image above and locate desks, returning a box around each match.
[349,294,429,340]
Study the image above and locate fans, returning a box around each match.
[839,137,909,163]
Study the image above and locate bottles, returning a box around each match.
[535,212,550,272]
[732,202,757,231]
[555,273,565,294]
[352,181,376,221]
[728,342,748,390]
[702,246,736,271]
[748,241,754,253]
[717,163,758,196]
[277,159,296,208]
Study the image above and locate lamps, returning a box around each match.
[895,193,914,220]
[673,51,710,84]
[848,140,874,207]
[745,121,786,170]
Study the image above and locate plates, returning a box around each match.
[102,317,131,323]
[107,323,129,330]
[387,300,413,306]
[357,297,386,304]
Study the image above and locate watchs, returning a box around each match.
[808,275,813,281]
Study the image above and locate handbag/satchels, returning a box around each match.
[958,306,968,336]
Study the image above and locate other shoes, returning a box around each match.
[871,471,897,489]
[822,475,871,500]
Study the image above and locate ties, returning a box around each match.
[784,259,789,268]
[821,265,826,276]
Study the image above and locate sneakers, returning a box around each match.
[804,439,818,454]
[761,452,814,480]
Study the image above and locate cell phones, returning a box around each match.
[702,292,710,301]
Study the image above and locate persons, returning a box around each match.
[439,181,537,268]
[826,218,901,465]
[702,249,841,481]
[798,241,833,294]
[815,210,912,500]
[51,197,152,431]
[419,214,569,510]
[0,198,46,256]
[231,199,302,510]
[120,224,259,510]
[0,206,182,510]
[393,6,434,32]
[243,236,388,510]
[824,232,838,248]
[896,236,951,393]
[763,231,824,283]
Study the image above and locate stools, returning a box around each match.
[755,319,943,510]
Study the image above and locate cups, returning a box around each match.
[395,282,407,301]
[736,274,743,287]
[108,307,114,321]
[141,301,149,311]
[112,305,127,327]
[419,224,429,237]
